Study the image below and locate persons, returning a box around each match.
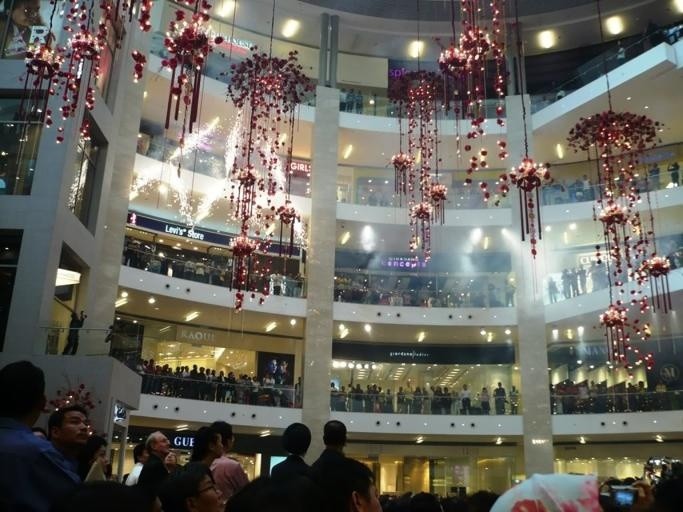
[61,308,88,356]
[354,89,364,114]
[616,41,631,64]
[1,360,682,511]
[122,235,305,298]
[346,89,358,111]
[548,234,682,304]
[338,87,348,112]
[368,152,681,210]
[642,18,664,49]
[334,270,516,307]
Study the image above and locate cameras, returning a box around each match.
[598,485,637,509]
